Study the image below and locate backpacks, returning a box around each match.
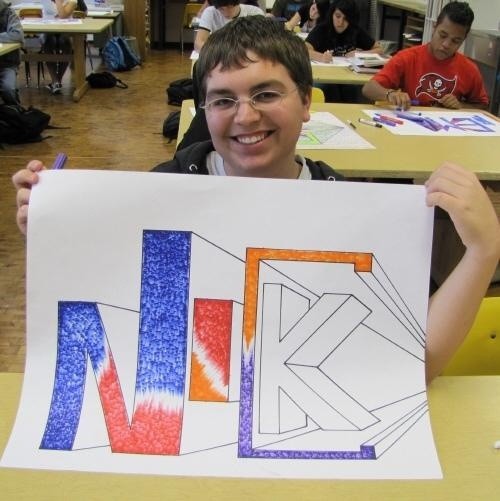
[166,79,193,106]
[0,103,51,144]
[162,110,181,143]
[85,71,127,88]
[102,33,141,71]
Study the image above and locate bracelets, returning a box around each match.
[385,88,396,102]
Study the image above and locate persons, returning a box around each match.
[306,3,384,64]
[11,13,500,385]
[193,1,265,54]
[42,0,78,94]
[0,0,26,105]
[362,0,490,112]
[286,0,329,34]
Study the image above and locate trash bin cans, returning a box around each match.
[125,36,140,61]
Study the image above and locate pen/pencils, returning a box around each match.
[327,50,334,64]
[52,153,67,170]
[359,99,424,128]
[347,119,356,129]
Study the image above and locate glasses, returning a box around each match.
[198,86,299,116]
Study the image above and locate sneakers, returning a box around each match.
[45,81,64,94]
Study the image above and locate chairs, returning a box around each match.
[440,298,499,375]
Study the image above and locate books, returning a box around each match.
[352,51,387,74]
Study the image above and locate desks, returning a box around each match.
[2,2,120,111]
[176,1,499,184]
[2,372,500,498]
